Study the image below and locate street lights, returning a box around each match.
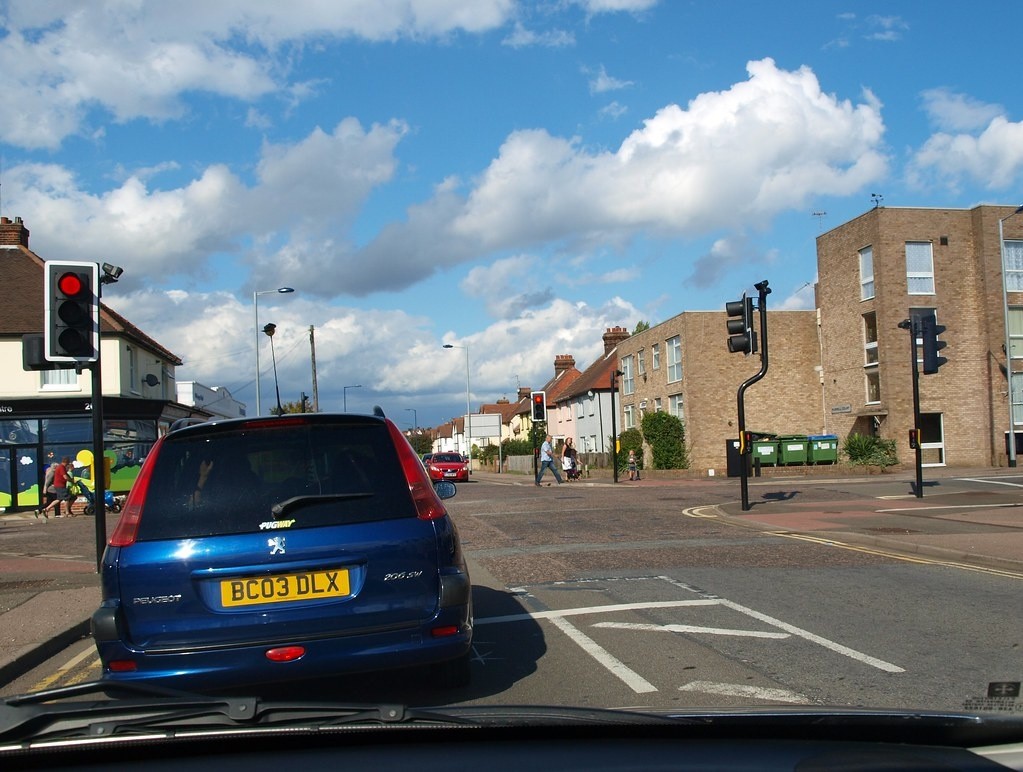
[344,385,362,412]
[588,389,603,453]
[405,409,416,430]
[253,288,295,417]
[443,344,472,476]
[261,322,286,416]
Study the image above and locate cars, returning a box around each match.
[421,452,470,482]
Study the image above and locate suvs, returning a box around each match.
[88,404,474,700]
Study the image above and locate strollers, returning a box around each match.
[557,458,582,482]
[76,479,121,515]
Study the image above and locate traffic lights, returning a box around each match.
[530,391,546,422]
[44,260,100,363]
[726,292,758,357]
[921,314,948,375]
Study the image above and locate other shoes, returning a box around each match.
[35,509,40,518]
[67,512,77,518]
[630,477,633,481]
[567,479,573,483]
[45,509,51,519]
[55,515,65,518]
[535,483,542,487]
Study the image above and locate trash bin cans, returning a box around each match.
[752,432,780,467]
[808,434,838,465]
[726,439,753,477]
[777,434,809,466]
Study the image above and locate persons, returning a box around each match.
[191,460,213,505]
[535,435,565,486]
[35,456,77,518]
[627,450,638,481]
[561,437,582,482]
